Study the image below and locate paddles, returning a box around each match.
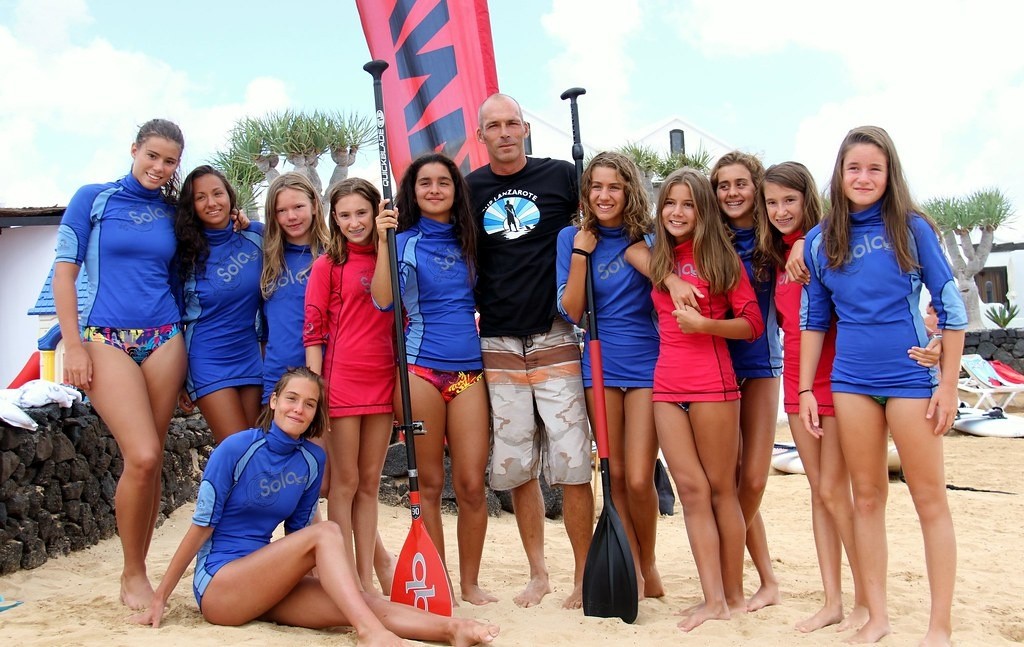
[362,59,454,616]
[559,87,639,624]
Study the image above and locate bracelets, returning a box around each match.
[796,389,814,395]
[571,247,590,256]
[798,237,806,240]
[927,330,941,340]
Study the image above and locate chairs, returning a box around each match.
[958,354,1024,418]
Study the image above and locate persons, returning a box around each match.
[51,120,189,611]
[179,92,869,632]
[925,302,942,336]
[799,126,968,647]
[130,367,500,647]
